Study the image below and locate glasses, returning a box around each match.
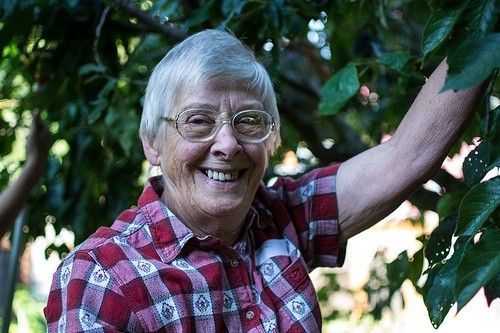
[162,109,275,144]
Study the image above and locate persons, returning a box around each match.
[45,29,495,333]
